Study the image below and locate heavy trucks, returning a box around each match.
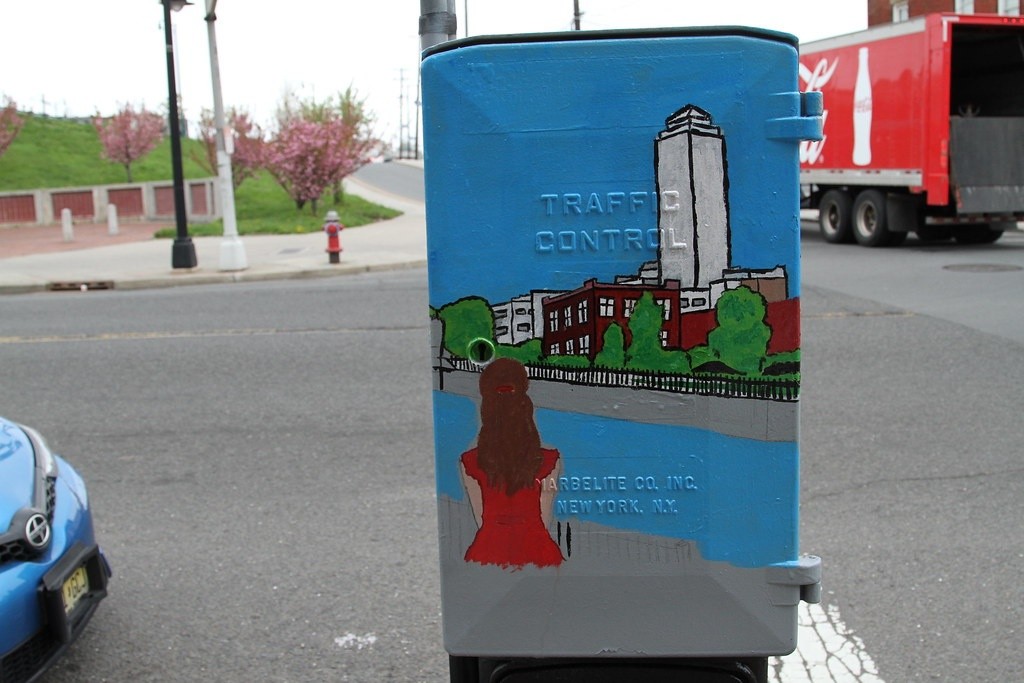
[799,13,1024,247]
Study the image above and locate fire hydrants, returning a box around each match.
[321,211,343,263]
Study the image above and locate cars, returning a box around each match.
[1,413,112,682]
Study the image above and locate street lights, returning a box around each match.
[159,0,198,268]
